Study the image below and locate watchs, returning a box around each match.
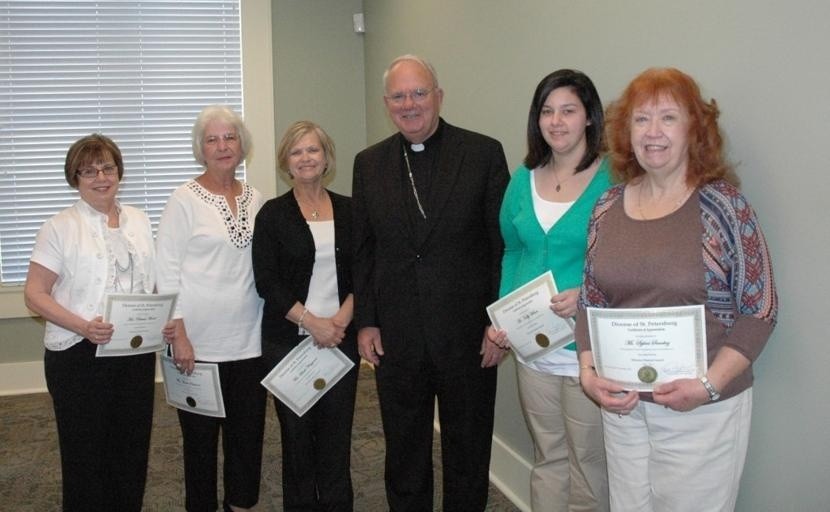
[699,376,720,401]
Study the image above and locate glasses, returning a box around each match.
[77,165,119,177]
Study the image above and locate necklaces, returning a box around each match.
[551,157,575,193]
[403,145,426,220]
[638,172,692,220]
[294,187,323,220]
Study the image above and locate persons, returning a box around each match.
[487,70,622,512]
[574,67,778,512]
[351,55,510,512]
[23,135,177,512]
[157,106,267,512]
[253,121,361,512]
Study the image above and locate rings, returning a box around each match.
[330,342,337,348]
[96,335,98,340]
[618,411,623,419]
[176,363,182,370]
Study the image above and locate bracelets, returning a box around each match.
[580,365,595,370]
[298,309,309,328]
